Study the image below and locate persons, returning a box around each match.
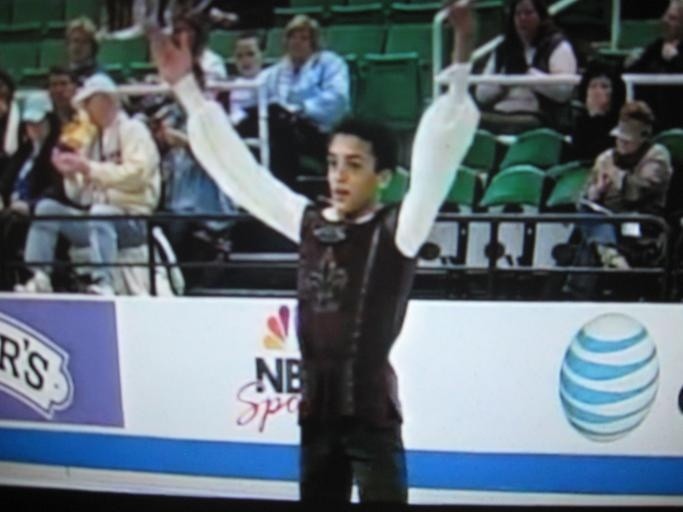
[0,0,350,294]
[550,101,675,302]
[569,60,629,163]
[149,0,482,512]
[618,0,682,135]
[475,0,582,134]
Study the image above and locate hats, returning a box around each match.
[70,72,117,109]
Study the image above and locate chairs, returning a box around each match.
[0,0,683,265]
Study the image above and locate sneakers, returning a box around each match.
[13,270,54,293]
[86,278,116,296]
